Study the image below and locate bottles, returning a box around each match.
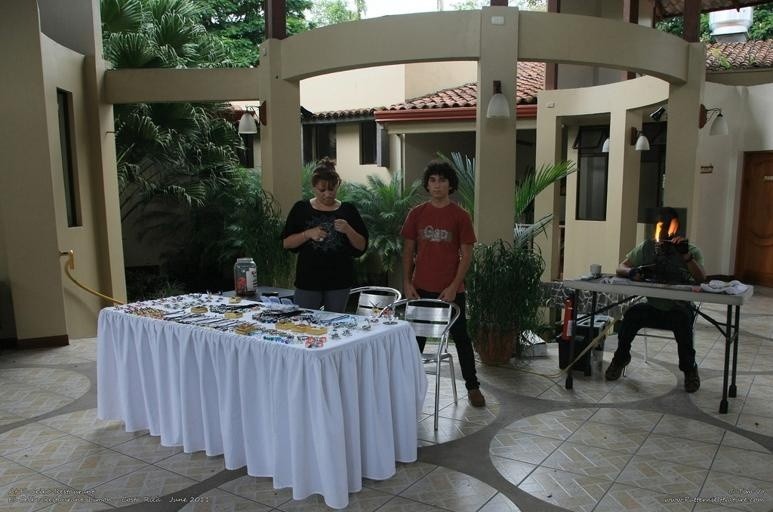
[233,257,256,297]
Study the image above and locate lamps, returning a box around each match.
[650,107,667,121]
[630,127,650,152]
[602,137,610,153]
[485,81,511,119]
[699,102,729,137]
[237,100,266,135]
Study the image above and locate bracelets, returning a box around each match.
[300,231,306,242]
[684,253,695,262]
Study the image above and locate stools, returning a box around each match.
[621,320,673,376]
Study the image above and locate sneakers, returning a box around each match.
[685,362,700,393]
[468,389,485,407]
[605,351,631,380]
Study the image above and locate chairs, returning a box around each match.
[378,298,460,433]
[319,286,402,317]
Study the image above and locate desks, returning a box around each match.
[563,263,753,414]
[94,293,427,511]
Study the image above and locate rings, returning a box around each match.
[318,238,323,242]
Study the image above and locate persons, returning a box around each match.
[605,207,705,393]
[281,157,368,311]
[400,159,485,408]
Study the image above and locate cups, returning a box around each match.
[590,264,601,275]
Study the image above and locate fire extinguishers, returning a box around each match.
[562,291,576,341]
